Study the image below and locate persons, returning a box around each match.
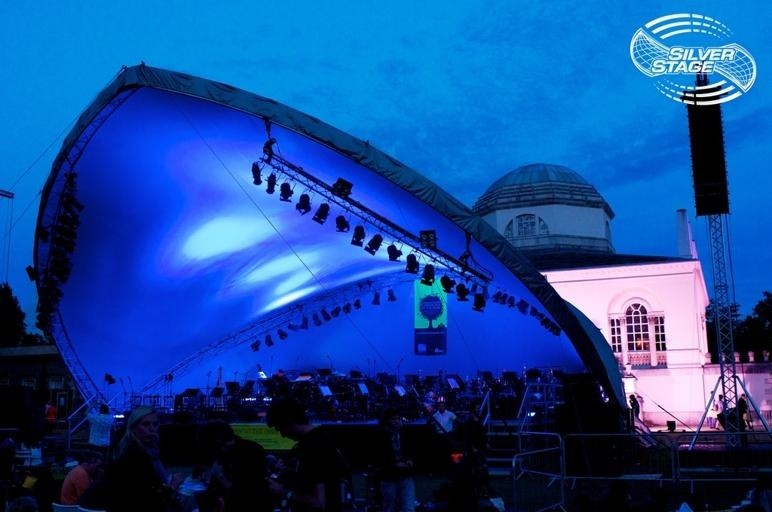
[1,431,24,479]
[366,407,418,511]
[629,394,646,427]
[737,393,747,432]
[193,417,274,512]
[44,401,60,432]
[86,404,120,464]
[708,391,718,429]
[460,399,489,474]
[430,401,463,447]
[69,405,189,511]
[263,396,343,510]
[715,394,724,430]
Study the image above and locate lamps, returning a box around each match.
[248,160,487,354]
